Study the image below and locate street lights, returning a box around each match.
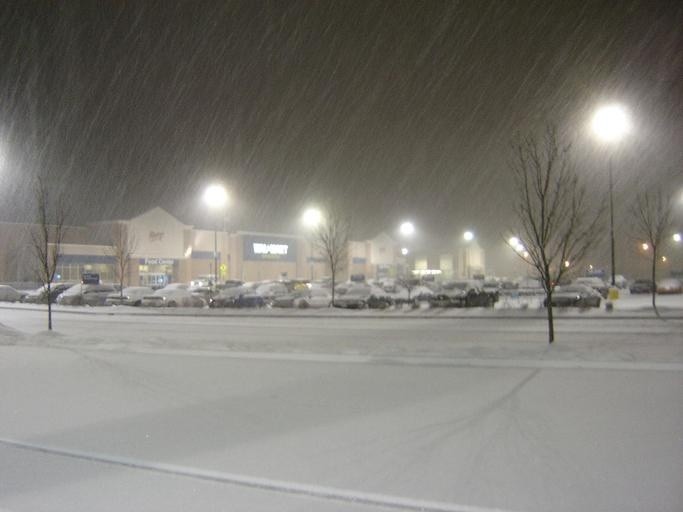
[203,186,228,293]
[465,232,473,280]
[400,222,415,290]
[301,208,323,283]
[593,105,616,287]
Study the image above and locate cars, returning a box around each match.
[434,280,481,301]
[0,286,27,304]
[334,280,387,307]
[543,275,624,309]
[629,274,680,294]
